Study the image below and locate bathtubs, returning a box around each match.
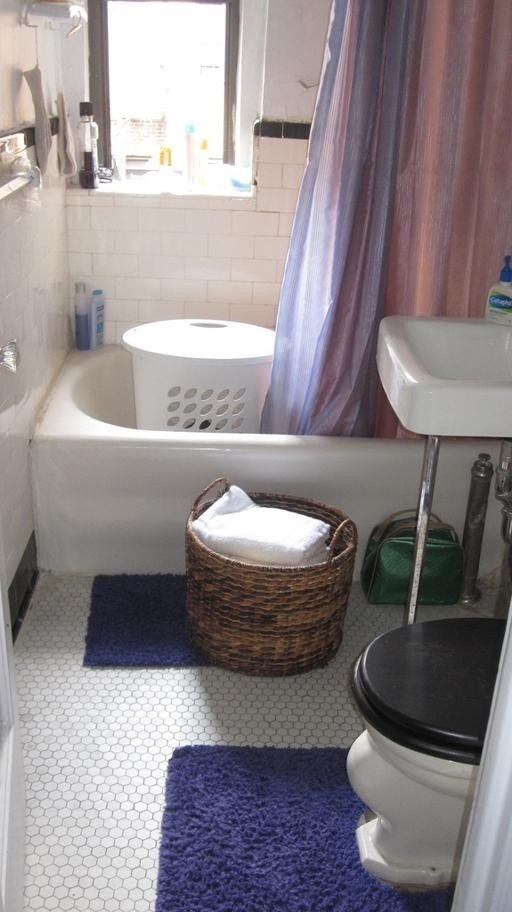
[27,335,512,576]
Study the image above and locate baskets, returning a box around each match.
[183,478,357,677]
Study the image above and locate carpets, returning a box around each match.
[154,744,457,911]
[84,573,213,667]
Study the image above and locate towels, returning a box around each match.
[191,484,332,567]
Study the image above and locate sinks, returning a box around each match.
[375,314,512,440]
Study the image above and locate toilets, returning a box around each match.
[342,617,511,895]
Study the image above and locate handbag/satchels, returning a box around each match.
[361,510,466,605]
